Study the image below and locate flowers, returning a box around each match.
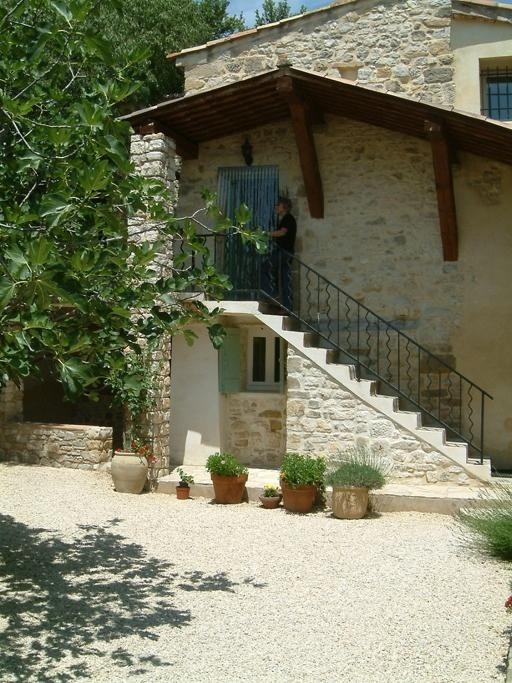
[113,431,158,465]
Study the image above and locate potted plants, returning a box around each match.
[176,444,393,521]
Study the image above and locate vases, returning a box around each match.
[110,450,149,493]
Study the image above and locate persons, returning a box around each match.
[259,196,297,313]
